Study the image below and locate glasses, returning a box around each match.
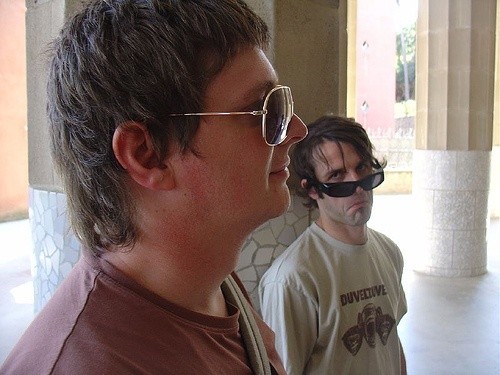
[309,158,387,197]
[160,83,296,147]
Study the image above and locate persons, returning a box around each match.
[0,1,311,375]
[258,114,414,375]
